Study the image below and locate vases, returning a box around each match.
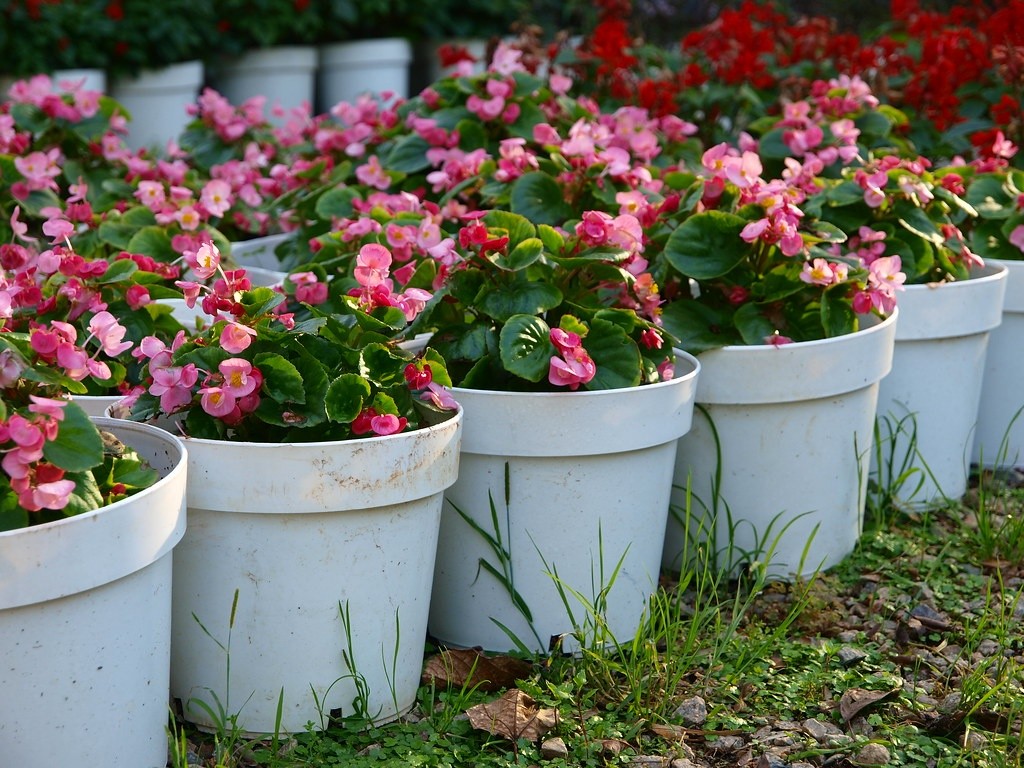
[426,345,701,658]
[105,397,463,738]
[863,257,1009,510]
[228,267,282,293]
[318,38,413,127]
[226,235,334,280]
[428,39,488,85]
[60,393,130,416]
[662,295,901,578]
[210,45,318,127]
[0,415,188,768]
[109,60,204,158]
[152,298,218,341]
[972,259,1024,467]
[0,69,106,108]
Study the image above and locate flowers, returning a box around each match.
[0,2,1024,534]
